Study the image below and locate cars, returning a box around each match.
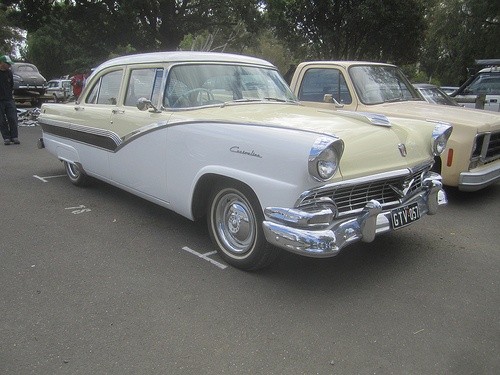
[10,63,47,95]
[362,84,460,108]
[197,69,287,104]
[439,86,459,95]
[455,68,500,112]
[39,51,453,273]
[290,62,500,193]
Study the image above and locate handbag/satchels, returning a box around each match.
[78,80,83,87]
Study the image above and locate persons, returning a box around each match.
[70,71,84,100]
[0,54,22,145]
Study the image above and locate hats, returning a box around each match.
[0,56,14,64]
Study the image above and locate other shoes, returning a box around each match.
[4,138,20,145]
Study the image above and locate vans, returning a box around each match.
[47,78,72,104]
